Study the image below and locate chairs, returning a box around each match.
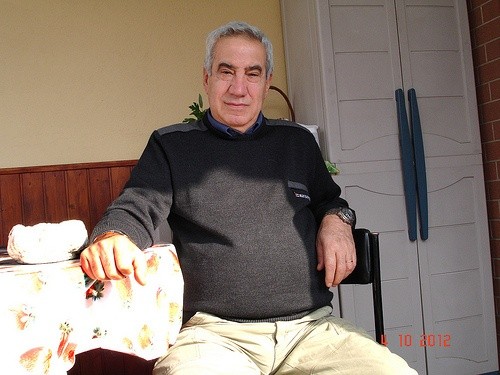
[337,228,385,347]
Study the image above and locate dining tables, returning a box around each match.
[0,243,185,375]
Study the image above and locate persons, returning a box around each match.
[80,18,423,372]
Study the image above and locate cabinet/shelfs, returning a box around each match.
[279,0,499,374]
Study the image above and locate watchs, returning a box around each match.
[322,205,356,226]
